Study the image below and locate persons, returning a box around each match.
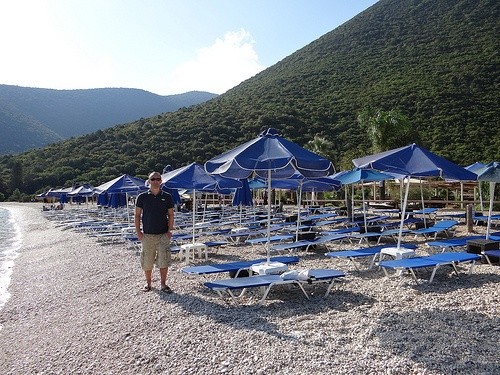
[135,172,174,294]
[55,204,63,210]
[180,201,190,211]
[43,206,49,211]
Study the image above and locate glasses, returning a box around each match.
[150,178,161,181]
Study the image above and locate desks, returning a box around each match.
[252,261,289,294]
[180,243,208,263]
[379,247,415,275]
[230,227,249,243]
[465,239,499,263]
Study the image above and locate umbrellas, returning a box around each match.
[35,174,149,228]
[144,162,243,261]
[204,128,500,266]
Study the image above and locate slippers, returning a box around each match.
[145,285,151,291]
[161,286,172,293]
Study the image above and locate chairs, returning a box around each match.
[47,205,500,310]
